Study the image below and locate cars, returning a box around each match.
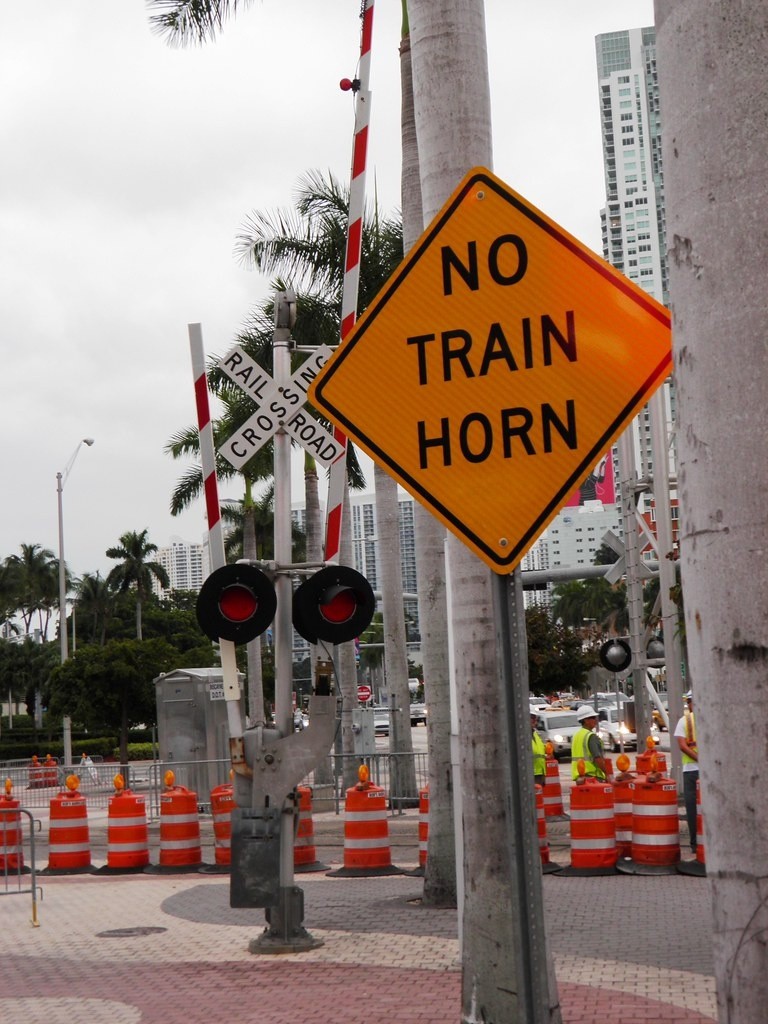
[410,700,426,728]
[375,712,389,736]
[529,690,689,759]
[293,708,309,731]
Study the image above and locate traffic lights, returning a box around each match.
[291,565,376,645]
[194,563,278,644]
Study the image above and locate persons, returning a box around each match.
[529,703,546,789]
[571,705,608,786]
[673,688,705,862]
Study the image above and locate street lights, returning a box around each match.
[72,591,88,660]
[54,436,95,777]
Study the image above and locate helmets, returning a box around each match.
[529,703,544,716]
[682,690,693,700]
[576,704,600,722]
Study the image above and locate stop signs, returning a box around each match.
[356,686,372,702]
